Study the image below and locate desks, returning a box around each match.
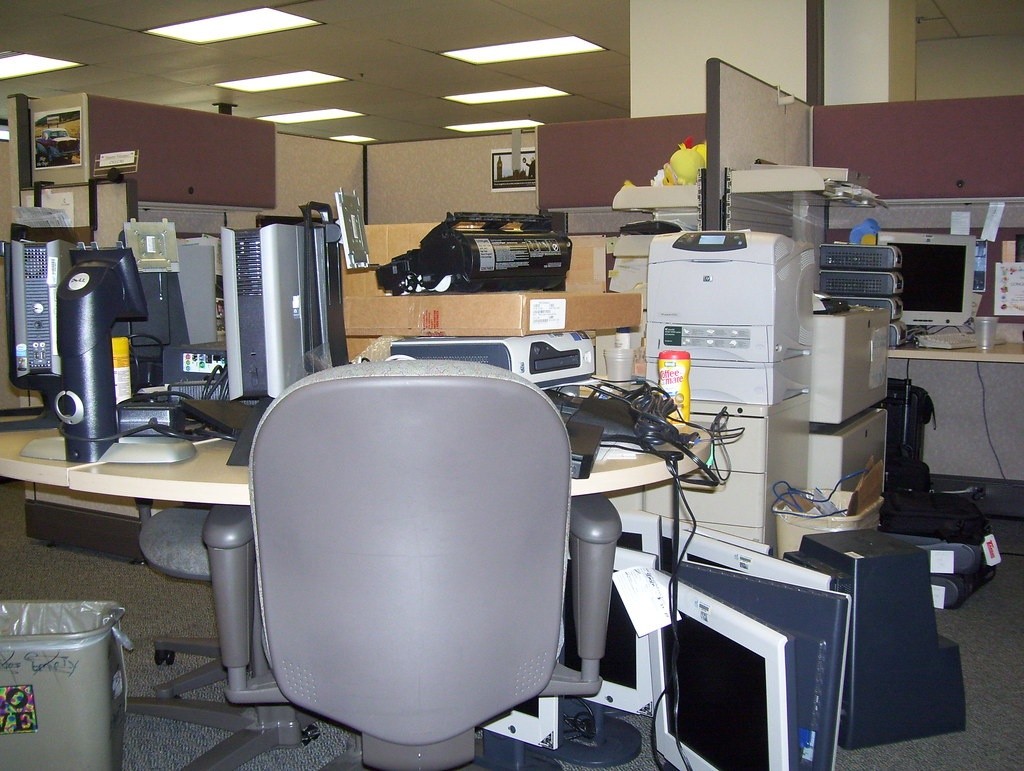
[1,422,709,566]
[884,339,1024,364]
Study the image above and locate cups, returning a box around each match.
[604,348,634,386]
[973,317,999,350]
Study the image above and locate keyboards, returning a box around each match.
[918,332,1006,350]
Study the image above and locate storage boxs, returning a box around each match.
[341,222,640,356]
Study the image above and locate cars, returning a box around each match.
[35,128,80,165]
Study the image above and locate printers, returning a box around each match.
[387,328,595,390]
[646,228,890,558]
[375,211,573,297]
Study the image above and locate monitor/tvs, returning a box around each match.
[877,230,976,335]
[470,509,851,771]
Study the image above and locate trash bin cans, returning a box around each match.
[772,487,885,559]
[0,599,128,771]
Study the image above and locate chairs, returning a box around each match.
[127,496,328,770]
[203,358,623,771]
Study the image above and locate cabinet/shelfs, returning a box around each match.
[628,392,813,560]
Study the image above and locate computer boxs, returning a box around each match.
[219,223,349,401]
[10,239,79,379]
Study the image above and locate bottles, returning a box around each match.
[658,350,691,426]
